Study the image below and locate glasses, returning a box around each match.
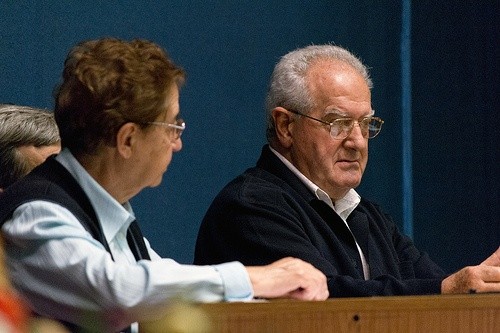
[287,108,384,139]
[147,118,186,141]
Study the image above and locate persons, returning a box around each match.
[0,38,330,333]
[192,45,500,294]
[0,103,61,194]
